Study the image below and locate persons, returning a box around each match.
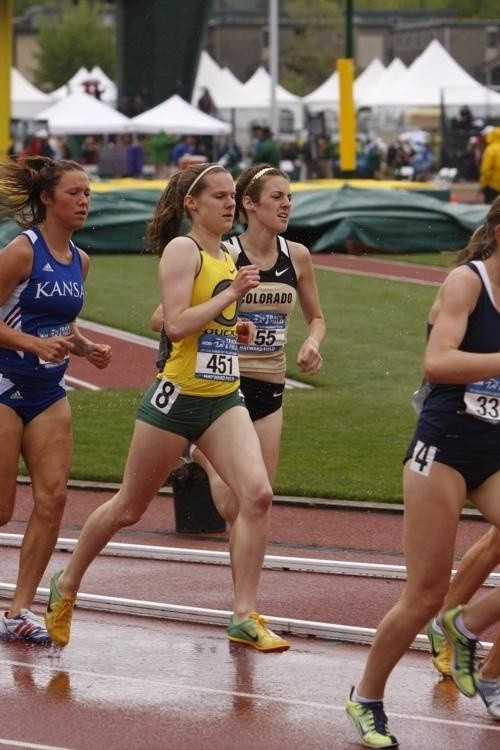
[0,156,114,649]
[43,160,292,656]
[8,127,71,169]
[148,163,328,591]
[339,194,500,750]
[79,134,196,181]
[420,523,500,723]
[478,124,500,205]
[217,104,500,182]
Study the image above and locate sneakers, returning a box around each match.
[345,686,398,750]
[425,606,500,720]
[1,608,53,645]
[227,612,290,652]
[45,570,77,647]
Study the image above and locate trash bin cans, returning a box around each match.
[173,466,226,534]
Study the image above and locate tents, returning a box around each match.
[33,90,233,150]
